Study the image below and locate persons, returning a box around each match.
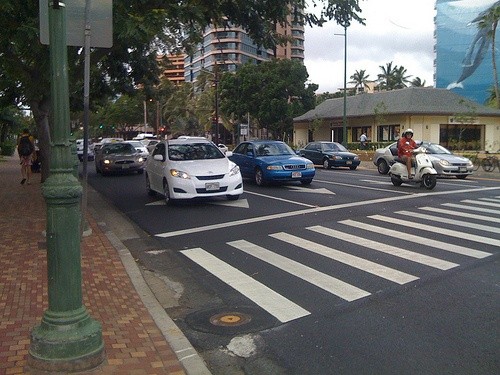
[360,133,367,150]
[399,129,417,180]
[17,128,37,185]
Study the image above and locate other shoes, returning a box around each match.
[20,177,26,184]
[408,176,413,179]
[27,180,32,184]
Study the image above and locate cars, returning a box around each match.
[142,135,244,207]
[75,131,229,177]
[299,141,362,170]
[228,140,316,187]
[372,140,475,179]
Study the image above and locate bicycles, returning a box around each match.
[468,150,500,173]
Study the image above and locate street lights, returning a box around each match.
[334,14,349,149]
[189,61,219,146]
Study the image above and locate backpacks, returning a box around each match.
[18,135,34,156]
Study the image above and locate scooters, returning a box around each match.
[387,141,438,190]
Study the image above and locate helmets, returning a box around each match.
[404,129,413,137]
